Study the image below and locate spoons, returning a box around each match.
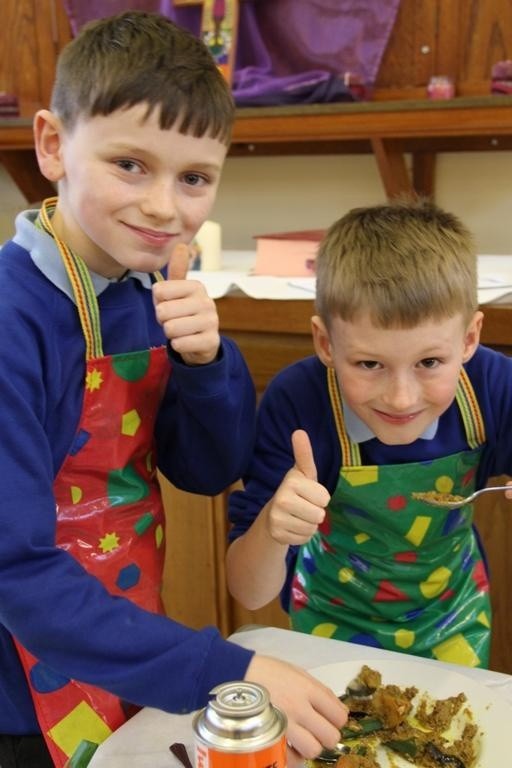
[287,741,351,762]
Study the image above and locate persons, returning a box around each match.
[0,9,350,768]
[222,203,512,670]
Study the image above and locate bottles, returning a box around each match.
[190,681,288,768]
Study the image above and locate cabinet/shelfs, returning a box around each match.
[155,296,512,635]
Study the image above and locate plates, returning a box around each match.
[305,661,512,768]
